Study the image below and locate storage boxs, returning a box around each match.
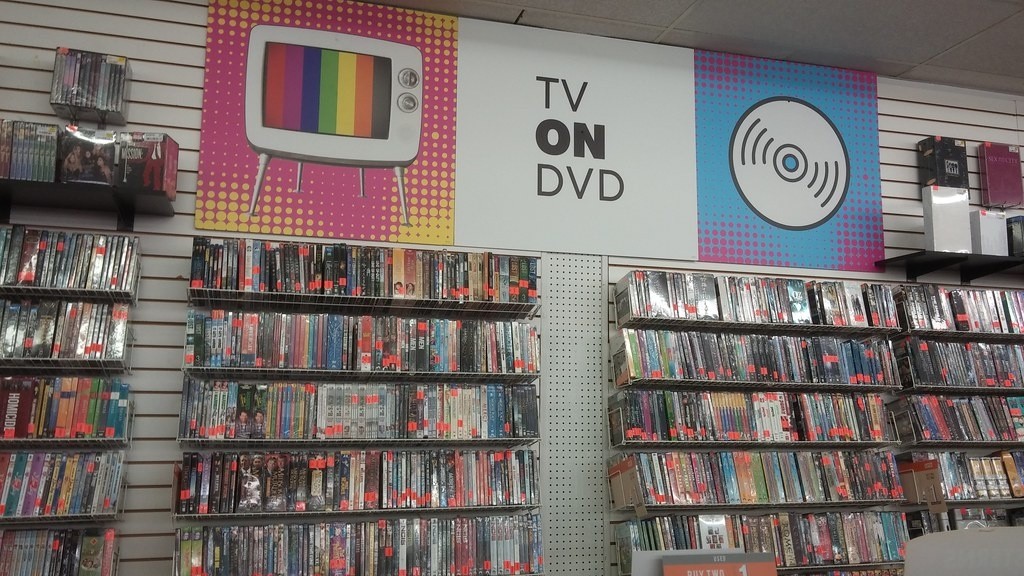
[917,135,1024,255]
[117,131,179,203]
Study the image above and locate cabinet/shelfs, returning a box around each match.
[0,283,176,576]
[170,287,544,525]
[607,280,1024,576]
